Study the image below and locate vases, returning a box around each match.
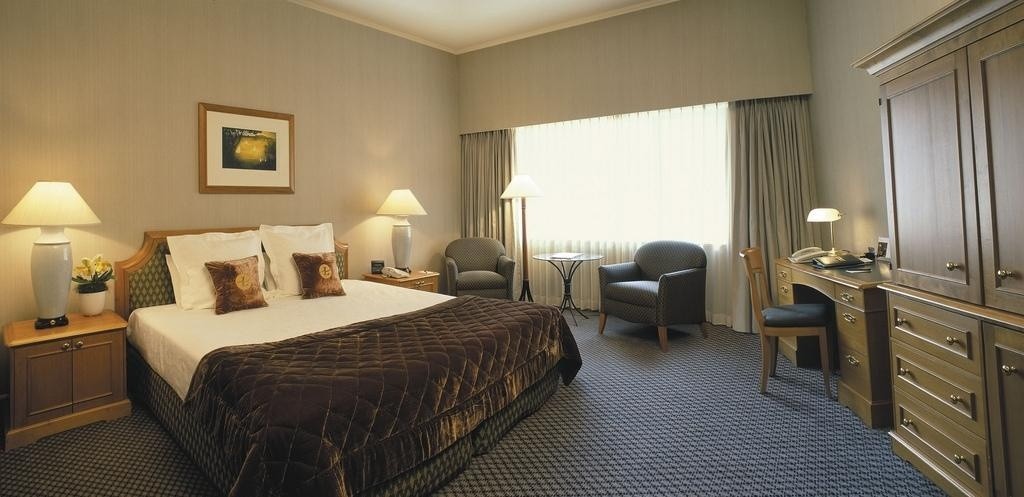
[79,291,105,316]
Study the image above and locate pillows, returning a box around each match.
[205,255,269,315]
[167,230,268,309]
[260,223,335,296]
[292,253,346,301]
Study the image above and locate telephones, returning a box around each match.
[381,267,409,278]
[792,247,828,262]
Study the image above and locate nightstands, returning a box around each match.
[362,268,440,293]
[2,309,134,452]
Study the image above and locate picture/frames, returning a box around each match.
[198,103,294,193]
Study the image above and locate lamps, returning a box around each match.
[376,190,428,273]
[807,208,849,256]
[500,174,545,302]
[1,182,102,329]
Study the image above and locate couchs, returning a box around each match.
[598,241,707,352]
[445,238,516,300]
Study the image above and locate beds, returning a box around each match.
[115,224,583,497]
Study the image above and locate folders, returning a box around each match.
[813,253,865,269]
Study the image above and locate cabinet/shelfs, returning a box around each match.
[851,0,1024,317]
[875,283,1024,497]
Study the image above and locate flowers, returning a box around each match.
[72,255,117,293]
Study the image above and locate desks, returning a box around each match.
[533,253,605,327]
[776,252,892,429]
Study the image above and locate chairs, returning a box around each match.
[739,246,831,399]
